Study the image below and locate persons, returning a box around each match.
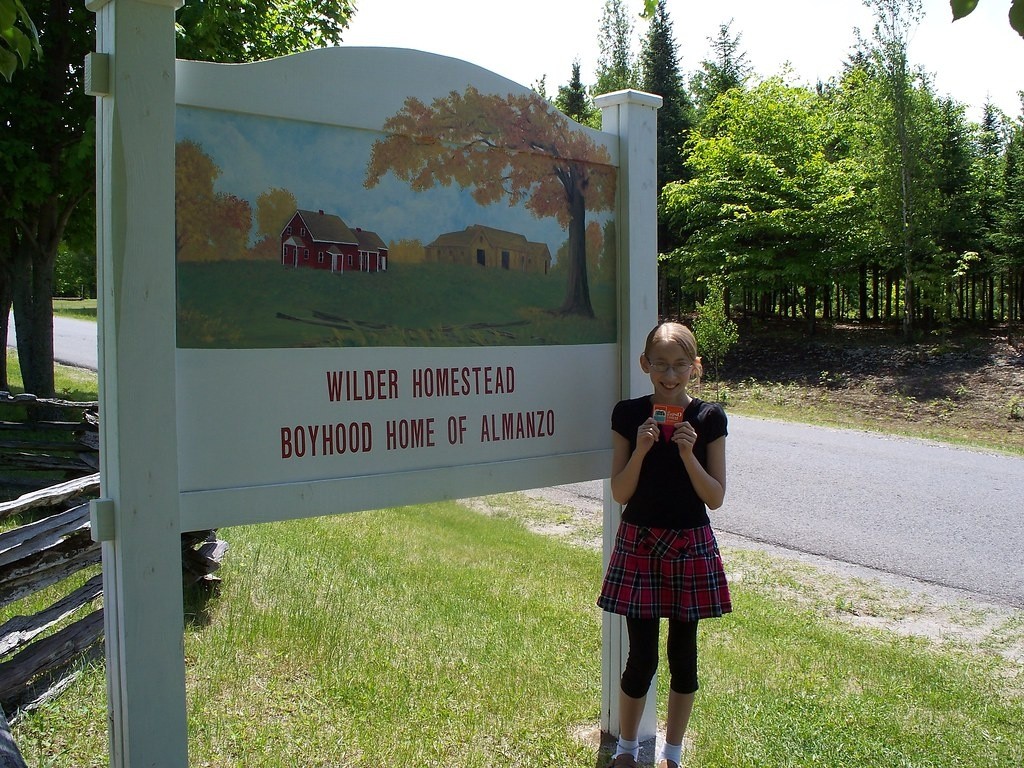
[595,322,733,768]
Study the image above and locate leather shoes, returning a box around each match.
[658,758,679,768]
[609,753,639,768]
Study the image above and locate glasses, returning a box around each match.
[642,352,693,373]
